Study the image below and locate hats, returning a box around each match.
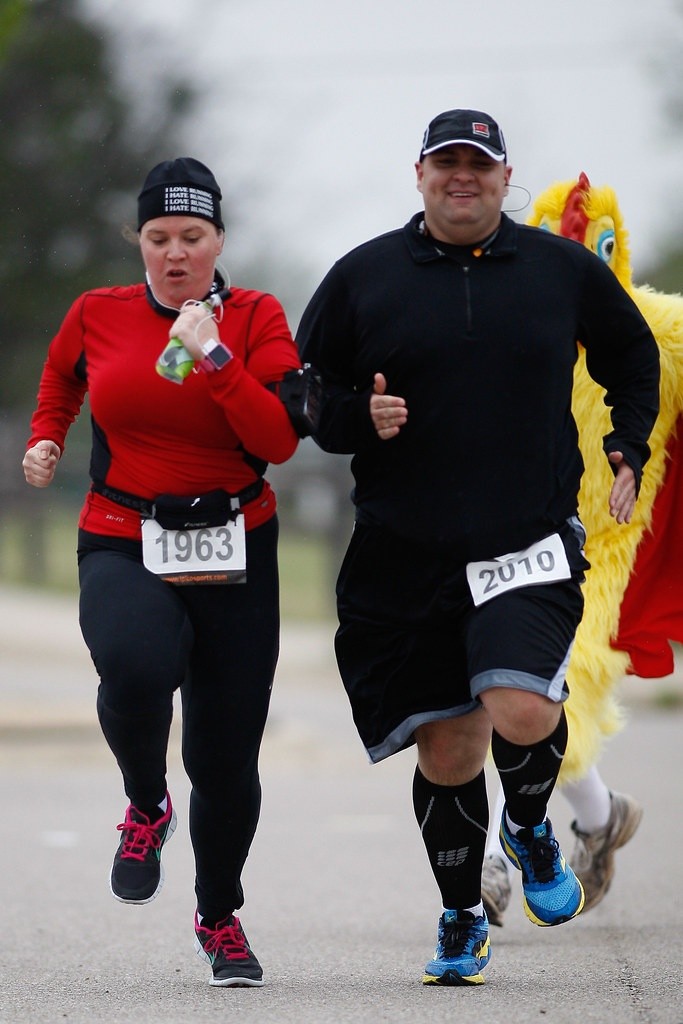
[137,157,225,231]
[419,109,507,166]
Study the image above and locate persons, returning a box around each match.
[478,169,683,926]
[21,159,304,988]
[295,109,662,983]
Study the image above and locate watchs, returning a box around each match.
[200,343,233,372]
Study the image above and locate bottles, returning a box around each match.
[155,297,213,386]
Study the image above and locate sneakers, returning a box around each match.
[481,852,513,928]
[194,909,265,988]
[499,801,584,928]
[112,792,178,903]
[563,788,643,914]
[422,911,492,984]
